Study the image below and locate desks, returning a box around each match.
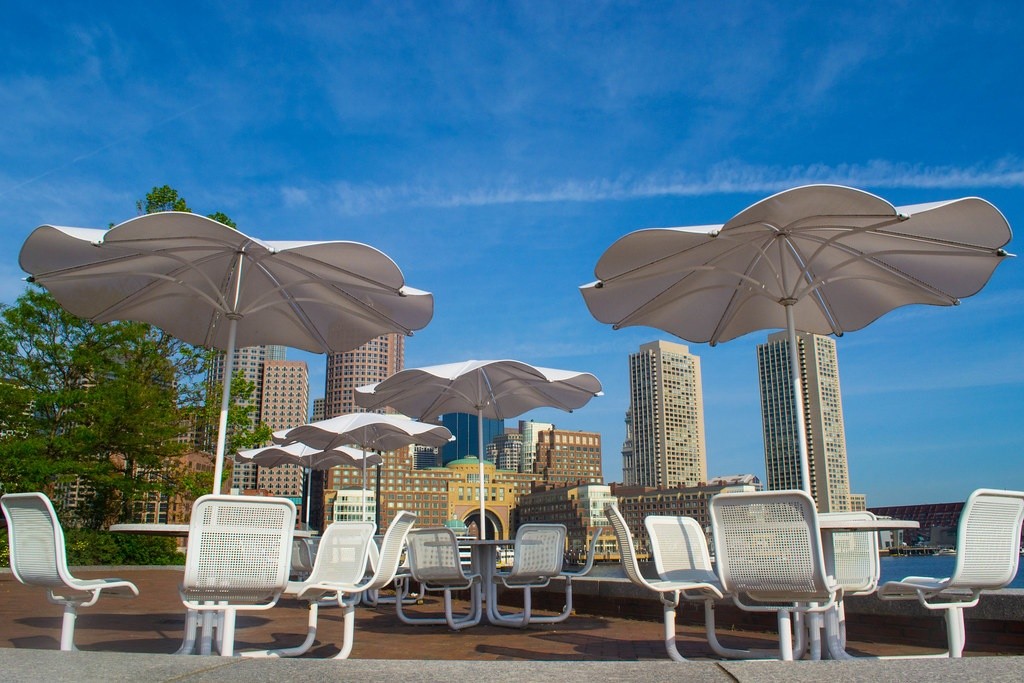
[310,536,387,601]
[107,524,310,653]
[421,540,542,619]
[705,520,924,656]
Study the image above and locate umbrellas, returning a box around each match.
[235,412,456,530]
[579,184,1017,491]
[354,359,602,540]
[18,210,434,495]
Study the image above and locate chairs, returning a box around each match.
[0,493,601,659]
[603,488,1024,661]
